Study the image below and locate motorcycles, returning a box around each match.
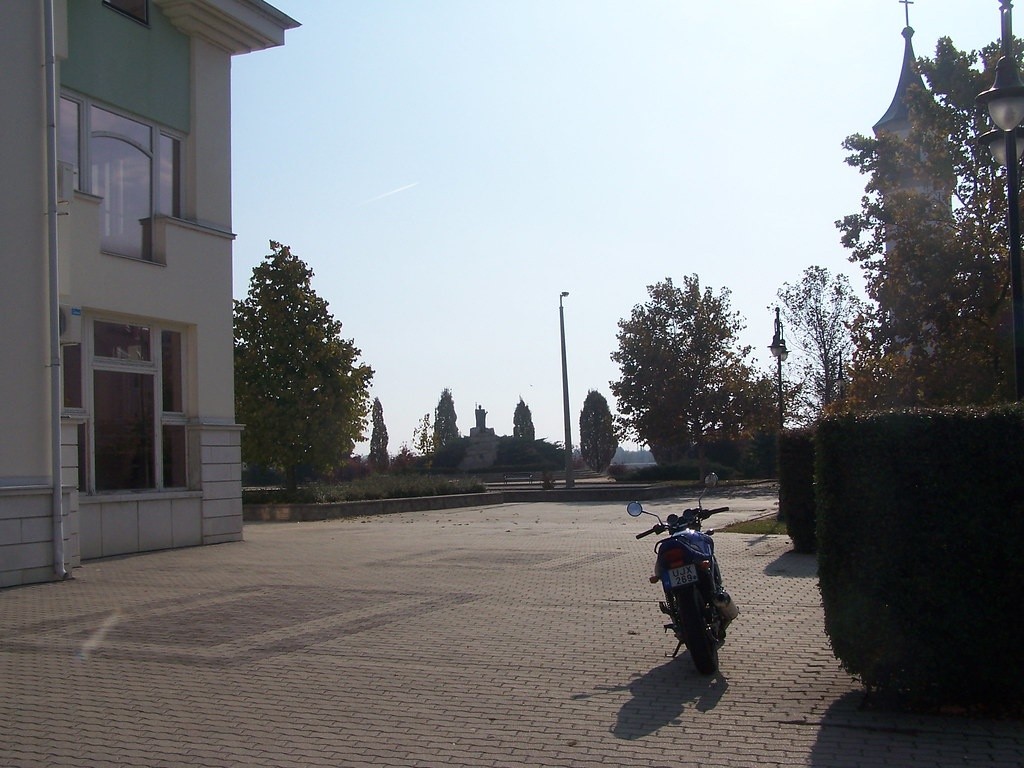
[627,472,738,677]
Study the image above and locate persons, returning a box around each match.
[529,472,533,485]
[475,405,488,428]
[503,474,507,486]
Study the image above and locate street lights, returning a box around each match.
[559,292,575,489]
[836,348,847,399]
[767,307,793,430]
